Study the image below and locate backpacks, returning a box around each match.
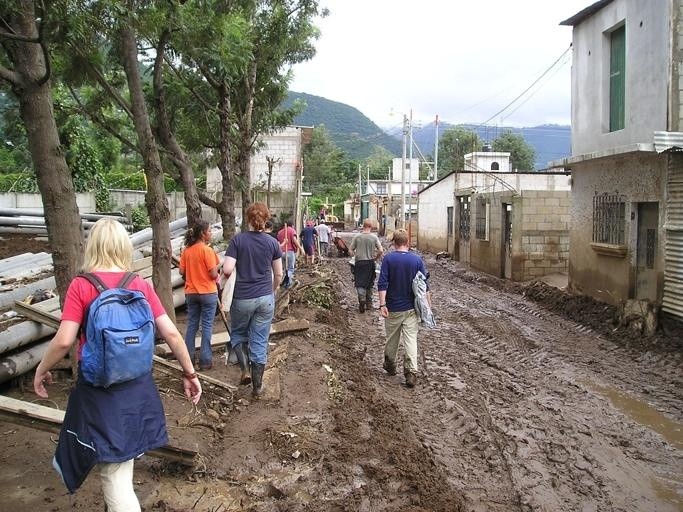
[77,270,155,388]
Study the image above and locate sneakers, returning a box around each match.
[359,298,370,312]
[383,362,397,375]
[406,373,416,385]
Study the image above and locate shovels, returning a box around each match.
[217,299,242,364]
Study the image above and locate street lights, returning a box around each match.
[263,154,280,209]
[294,191,314,260]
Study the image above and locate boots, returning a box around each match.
[249,361,266,396]
[233,342,251,385]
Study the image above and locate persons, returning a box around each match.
[299,220,319,268]
[355,215,360,227]
[178,219,218,369]
[303,209,308,227]
[377,228,431,386]
[316,220,330,257]
[349,220,383,313]
[34,218,202,512]
[328,222,336,242]
[277,219,303,290]
[319,208,326,219]
[264,221,288,247]
[222,202,284,400]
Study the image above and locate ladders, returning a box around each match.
[280,222,290,288]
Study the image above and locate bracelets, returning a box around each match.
[181,370,196,378]
[379,303,387,309]
